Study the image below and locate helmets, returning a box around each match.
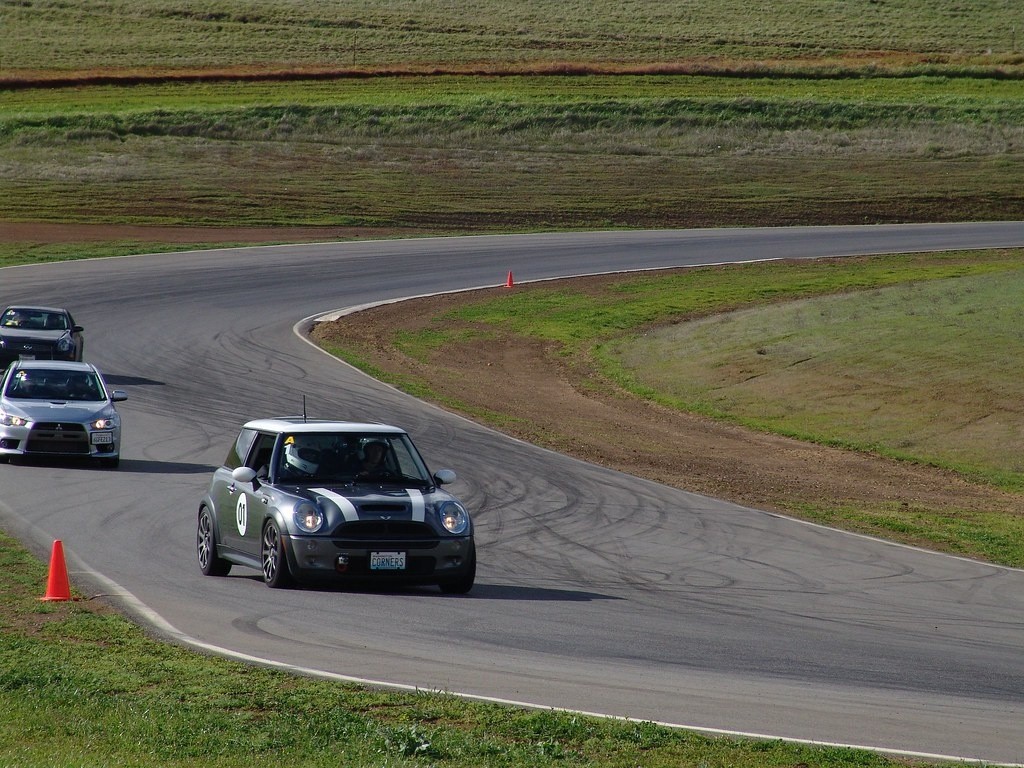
[358,436,390,465]
[18,374,32,389]
[285,436,321,475]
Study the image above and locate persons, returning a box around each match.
[67,374,90,398]
[14,372,38,395]
[44,314,60,327]
[335,438,402,483]
[12,312,30,326]
[257,437,323,485]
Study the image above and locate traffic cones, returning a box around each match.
[39,539,78,602]
[504,270,516,288]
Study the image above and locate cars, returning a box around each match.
[1,359,127,466]
[196,395,477,595]
[0,305,84,372]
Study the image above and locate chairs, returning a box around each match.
[43,316,59,326]
[298,448,318,463]
